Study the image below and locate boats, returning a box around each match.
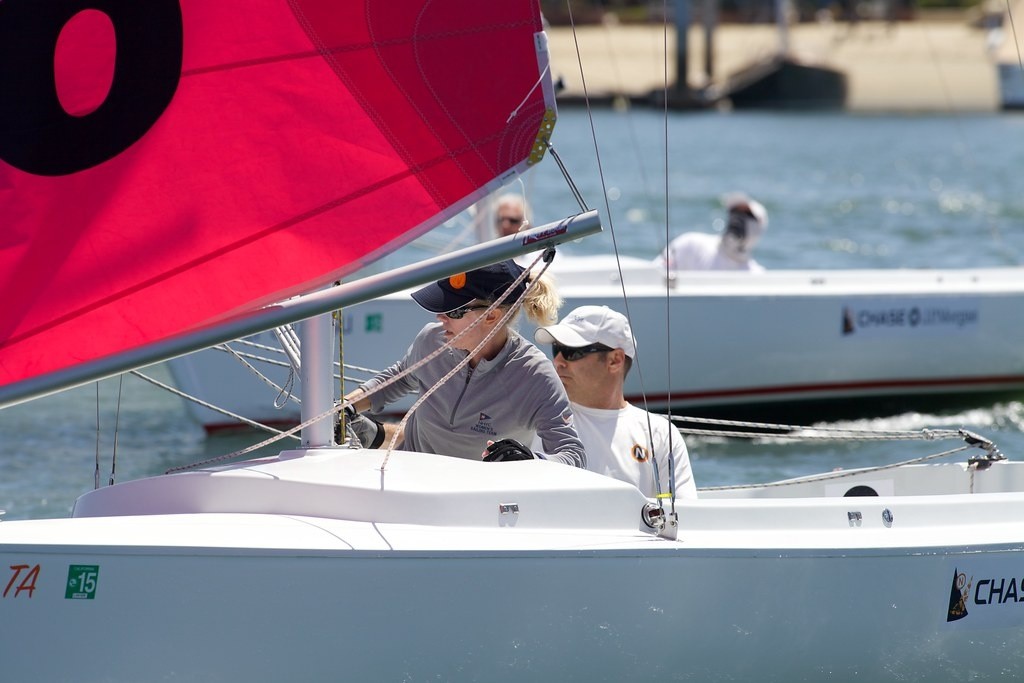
[638,1,848,116]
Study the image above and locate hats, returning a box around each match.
[541,304,638,359]
[410,258,531,314]
[727,198,768,226]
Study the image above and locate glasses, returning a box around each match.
[445,304,505,319]
[495,216,521,224]
[550,342,614,361]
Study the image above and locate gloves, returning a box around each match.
[332,397,385,449]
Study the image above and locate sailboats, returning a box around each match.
[165,196,1024,437]
[0,0,1024,682]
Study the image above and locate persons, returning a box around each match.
[335,259,697,499]
[656,195,767,271]
[495,194,559,259]
